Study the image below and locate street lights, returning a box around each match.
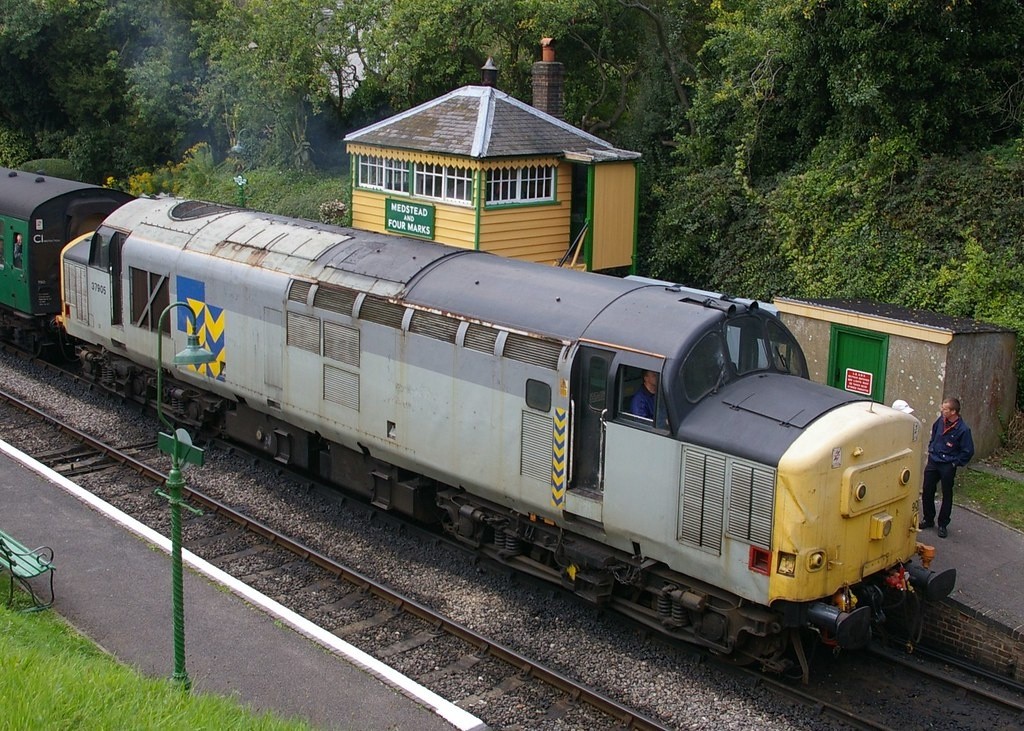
[231,128,254,208]
[154,297,222,694]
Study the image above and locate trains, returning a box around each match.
[0,165,961,680]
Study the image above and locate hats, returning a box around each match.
[892,399,914,414]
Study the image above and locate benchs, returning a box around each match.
[0,530,57,614]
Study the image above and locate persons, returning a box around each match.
[14,234,22,269]
[630,369,669,430]
[892,399,914,414]
[919,397,975,539]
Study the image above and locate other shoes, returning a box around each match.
[938,526,947,537]
[918,520,935,529]
